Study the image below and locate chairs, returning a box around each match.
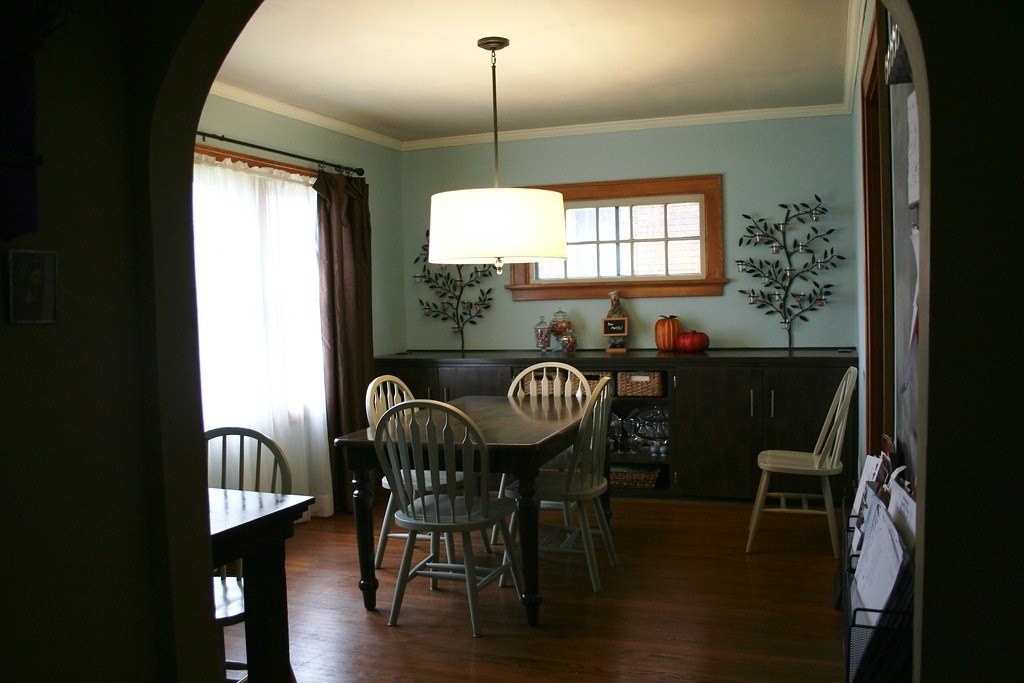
[375,398,528,642]
[745,364,858,559]
[204,427,291,683]
[498,375,618,596]
[365,374,492,570]
[491,364,592,548]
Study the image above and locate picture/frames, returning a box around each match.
[602,318,629,336]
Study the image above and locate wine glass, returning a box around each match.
[623,421,637,454]
[614,419,624,454]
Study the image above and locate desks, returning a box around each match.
[335,394,616,625]
[208,484,314,683]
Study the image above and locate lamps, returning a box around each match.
[427,37,568,275]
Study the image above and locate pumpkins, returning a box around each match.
[677,329,710,352]
[654,315,683,351]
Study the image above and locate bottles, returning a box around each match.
[533,316,553,354]
[551,307,572,352]
[560,328,577,354]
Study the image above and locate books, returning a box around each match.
[849,433,917,683]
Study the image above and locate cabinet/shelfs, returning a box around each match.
[371,362,858,506]
